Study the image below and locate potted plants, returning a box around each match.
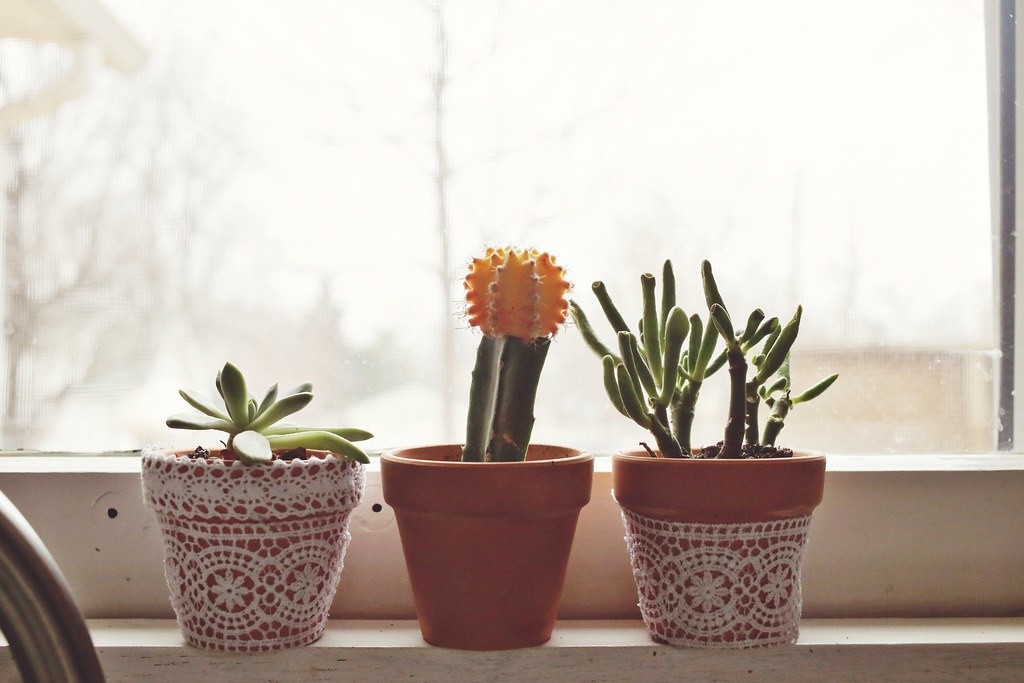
[380,247,595,652]
[569,260,837,649]
[141,364,372,651]
